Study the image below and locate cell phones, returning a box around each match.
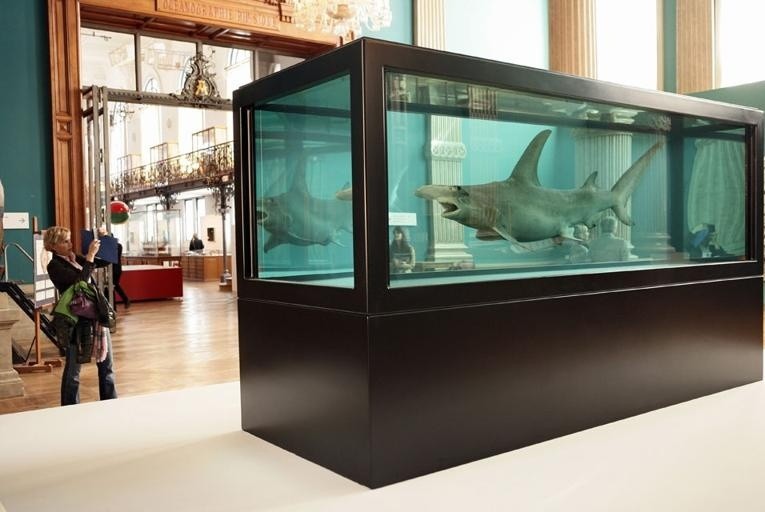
[92,228,100,239]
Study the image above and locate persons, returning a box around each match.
[389,226,415,273]
[42,225,120,407]
[112,234,129,310]
[690,225,727,258]
[569,224,590,262]
[590,216,630,261]
[189,233,204,251]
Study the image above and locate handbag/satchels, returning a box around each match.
[69,290,95,319]
[87,275,117,328]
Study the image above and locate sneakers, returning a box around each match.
[123,297,130,308]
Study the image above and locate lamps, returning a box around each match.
[290,0,392,41]
